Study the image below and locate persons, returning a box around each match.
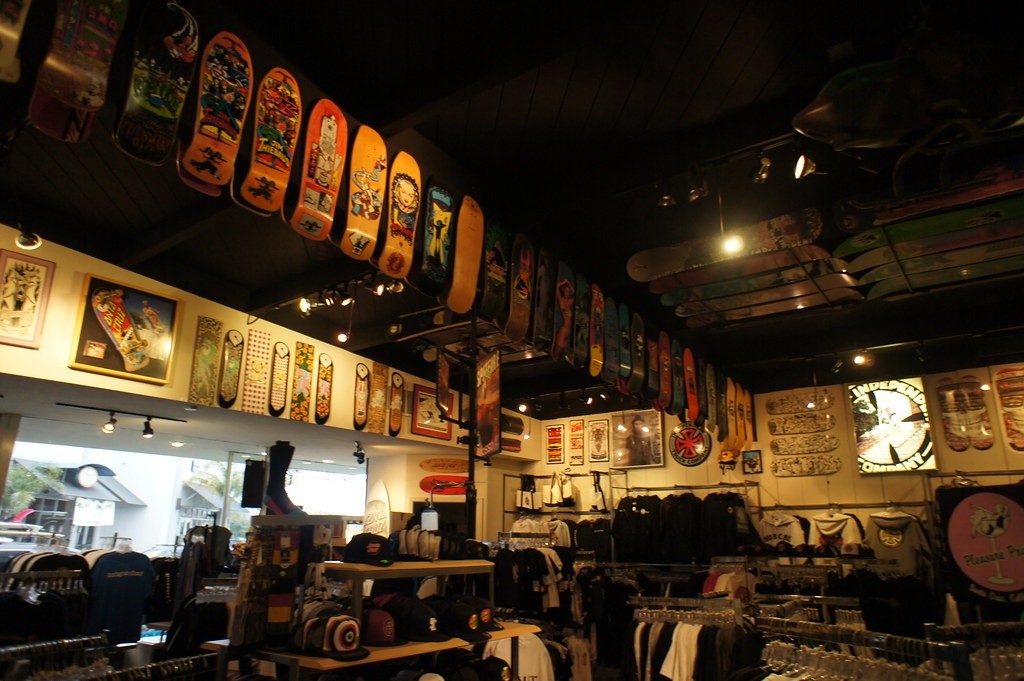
[626,415,652,464]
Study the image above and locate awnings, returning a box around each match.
[184,480,249,514]
[14,458,146,505]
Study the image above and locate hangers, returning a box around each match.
[33,531,132,555]
[498,531,552,550]
[631,595,735,627]
[0,633,209,681]
[712,556,745,573]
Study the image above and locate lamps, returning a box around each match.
[385,279,404,294]
[751,157,771,185]
[600,391,611,401]
[352,440,365,465]
[299,297,318,313]
[688,180,709,203]
[655,194,677,208]
[855,348,875,367]
[99,411,117,435]
[578,387,593,405]
[12,222,42,251]
[142,417,155,438]
[793,154,818,181]
[337,285,356,343]
[363,281,384,298]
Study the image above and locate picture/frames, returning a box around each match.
[611,409,665,470]
[69,270,184,386]
[742,450,764,474]
[545,424,565,464]
[569,419,584,466]
[410,385,454,443]
[0,249,57,350]
[588,419,610,462]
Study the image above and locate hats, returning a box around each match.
[289,526,514,680]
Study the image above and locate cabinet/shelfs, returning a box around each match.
[147,557,543,681]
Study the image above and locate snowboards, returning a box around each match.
[764,391,842,477]
[935,374,995,453]
[419,457,470,473]
[0,0,754,451]
[420,475,468,495]
